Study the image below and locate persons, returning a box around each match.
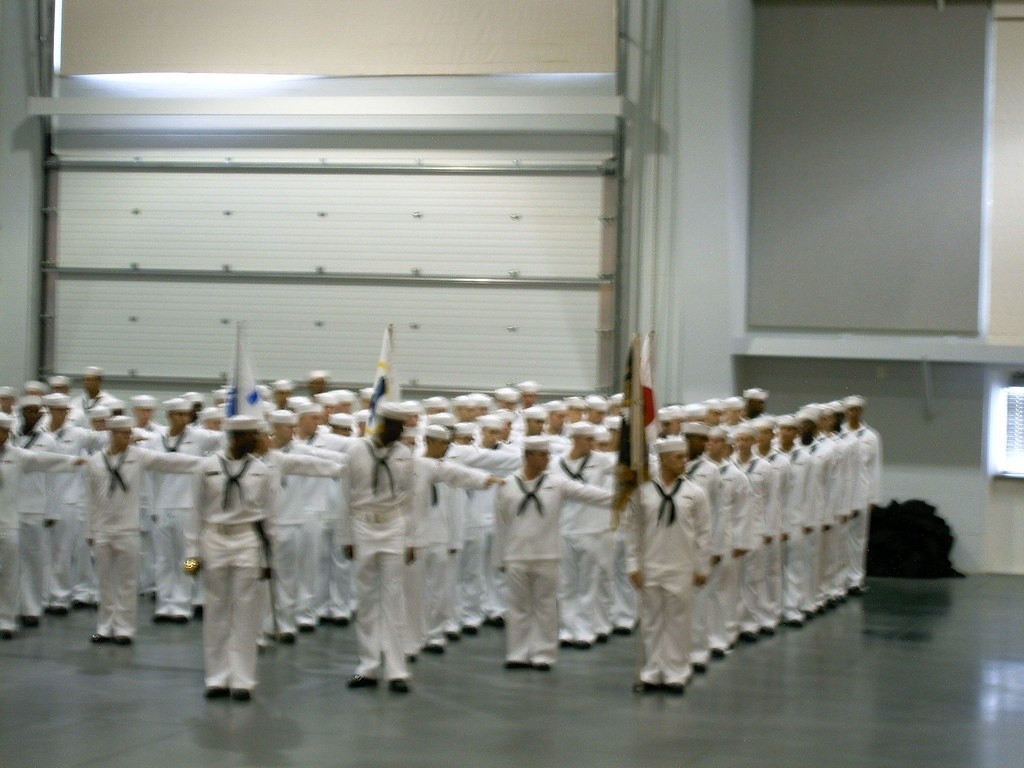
[0,366,881,699]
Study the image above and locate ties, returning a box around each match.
[684,449,802,481]
[560,452,592,484]
[512,472,548,518]
[164,428,186,454]
[17,432,41,453]
[98,446,131,497]
[365,438,399,496]
[428,480,440,509]
[218,453,251,513]
[653,479,684,528]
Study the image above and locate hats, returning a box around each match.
[653,435,687,453]
[0,366,868,450]
[424,423,454,445]
[521,434,553,453]
[374,396,412,424]
[226,413,263,434]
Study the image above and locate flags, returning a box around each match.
[225,333,262,419]
[613,332,655,527]
[366,329,394,437]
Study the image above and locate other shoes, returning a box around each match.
[636,675,686,698]
[0,585,862,674]
[502,657,552,672]
[346,669,415,698]
[200,686,252,706]
